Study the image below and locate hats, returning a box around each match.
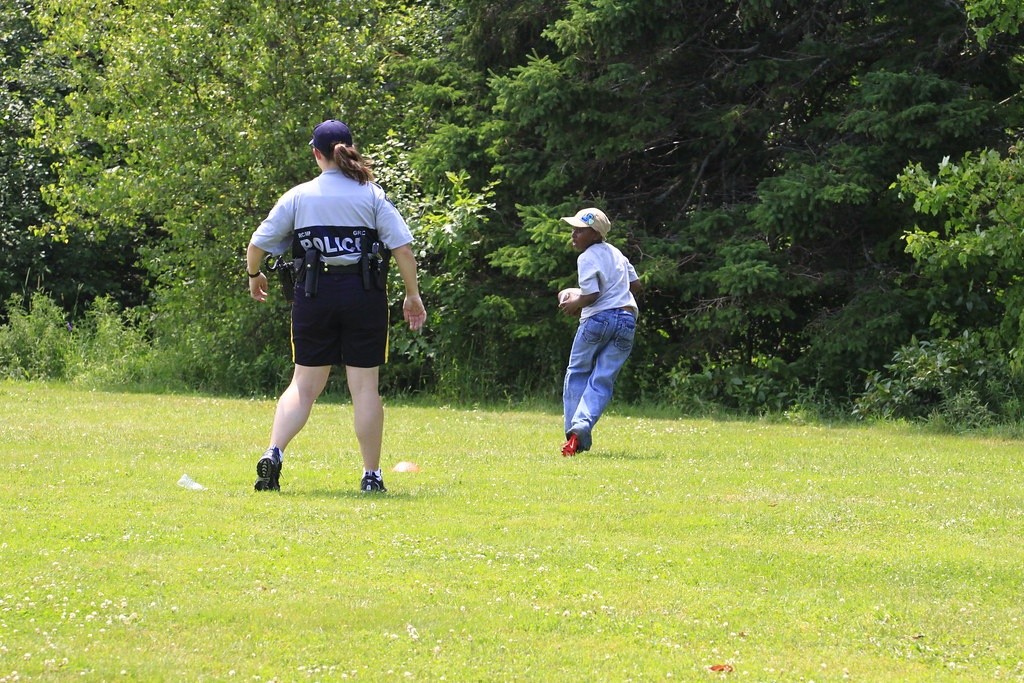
[309,120,353,153]
[561,207,612,238]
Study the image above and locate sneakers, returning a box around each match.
[360,469,388,494]
[561,432,579,457]
[254,446,281,492]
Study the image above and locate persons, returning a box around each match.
[556,208,642,455]
[247,119,427,495]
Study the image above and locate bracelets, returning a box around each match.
[247,269,261,277]
